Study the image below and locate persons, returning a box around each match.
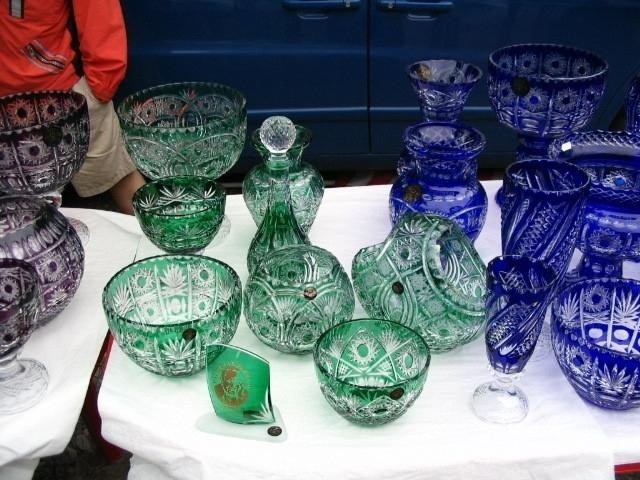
[1,0,150,215]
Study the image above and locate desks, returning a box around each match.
[0,206,143,480]
[97,176,640,479]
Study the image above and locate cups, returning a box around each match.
[129,174,226,255]
[352,215,489,355]
[407,57,484,123]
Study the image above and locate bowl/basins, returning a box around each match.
[549,277,638,414]
[102,257,244,376]
[311,317,430,433]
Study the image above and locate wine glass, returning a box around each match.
[502,159,593,362]
[117,78,246,249]
[0,257,52,416]
[548,129,640,296]
[1,93,94,252]
[486,42,610,208]
[471,252,556,427]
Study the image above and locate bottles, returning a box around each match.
[242,245,355,356]
[389,122,488,243]
[245,126,326,235]
[1,193,86,332]
